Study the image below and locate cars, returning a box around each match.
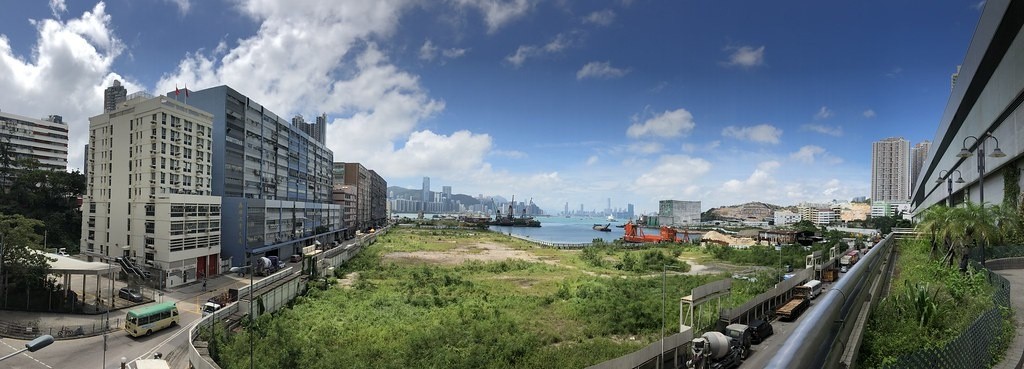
[279,261,285,268]
[119,287,143,302]
[841,266,847,272]
[57,252,70,258]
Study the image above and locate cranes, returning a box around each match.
[509,195,514,215]
[491,197,502,217]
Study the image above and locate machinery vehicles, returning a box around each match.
[251,254,279,277]
[686,324,752,369]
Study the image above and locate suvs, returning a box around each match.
[748,320,773,344]
[291,255,301,262]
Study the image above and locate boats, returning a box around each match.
[593,223,611,232]
[432,215,541,226]
[606,214,618,222]
[615,217,689,242]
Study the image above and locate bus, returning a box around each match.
[125,301,179,337]
[803,280,822,300]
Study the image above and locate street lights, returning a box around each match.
[956,135,1006,267]
[935,170,966,208]
[662,266,681,369]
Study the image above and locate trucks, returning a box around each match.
[200,292,238,319]
[776,286,810,318]
[840,250,859,268]
